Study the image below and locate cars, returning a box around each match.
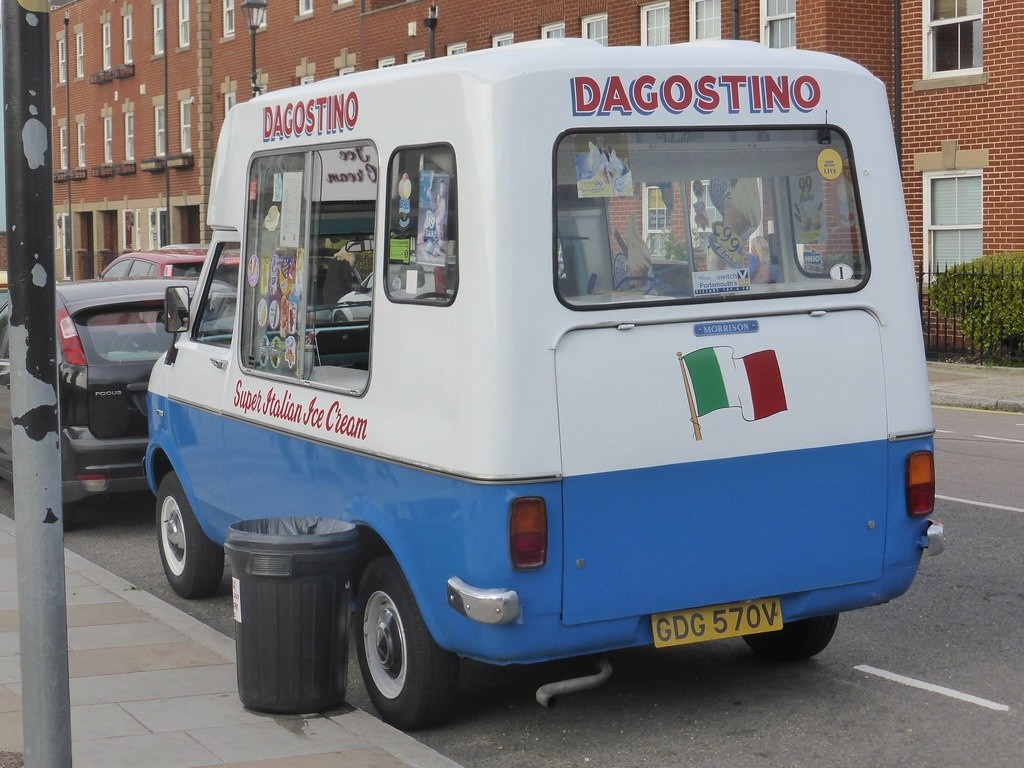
[0,243,467,528]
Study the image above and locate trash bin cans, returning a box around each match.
[223,516,359,715]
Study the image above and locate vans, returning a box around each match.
[142,33,936,730]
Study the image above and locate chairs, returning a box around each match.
[92,331,172,360]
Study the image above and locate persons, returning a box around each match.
[747,235,770,284]
[323,246,356,304]
[418,180,446,255]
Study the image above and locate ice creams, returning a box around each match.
[722,177,762,243]
[587,136,628,189]
[613,213,653,290]
[792,200,825,243]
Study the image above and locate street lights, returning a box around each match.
[240,0,268,99]
